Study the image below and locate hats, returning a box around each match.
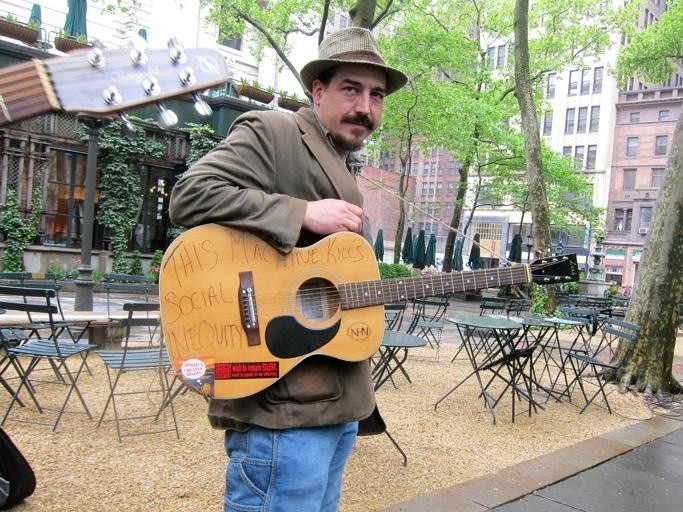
[300,28,407,97]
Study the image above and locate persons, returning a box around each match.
[168,26,410,511]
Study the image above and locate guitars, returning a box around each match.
[0,38,229,127]
[158,223,580,401]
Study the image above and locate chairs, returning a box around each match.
[368,289,643,427]
[1,264,209,445]
